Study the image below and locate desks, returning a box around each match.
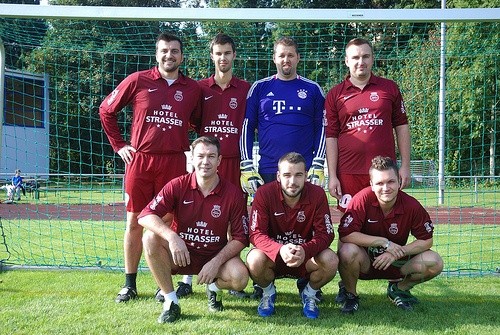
[22,178,116,197]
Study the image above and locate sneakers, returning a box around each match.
[297,277,322,303]
[158,301,182,323]
[251,285,276,300]
[176,281,193,299]
[301,287,320,319]
[116,287,138,301]
[155,289,165,300]
[229,289,250,298]
[388,283,413,311]
[342,292,360,313]
[387,283,419,304]
[336,281,346,302]
[258,288,276,316]
[206,283,224,311]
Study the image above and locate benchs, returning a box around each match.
[395,159,433,188]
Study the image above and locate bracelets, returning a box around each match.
[384,239,391,251]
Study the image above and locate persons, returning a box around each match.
[11,170,22,201]
[99,34,201,302]
[246,152,339,319]
[239,37,328,303]
[175,33,252,301]
[337,155,444,315]
[137,136,250,324]
[325,38,418,303]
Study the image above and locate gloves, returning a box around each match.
[240,160,264,194]
[307,158,326,189]
